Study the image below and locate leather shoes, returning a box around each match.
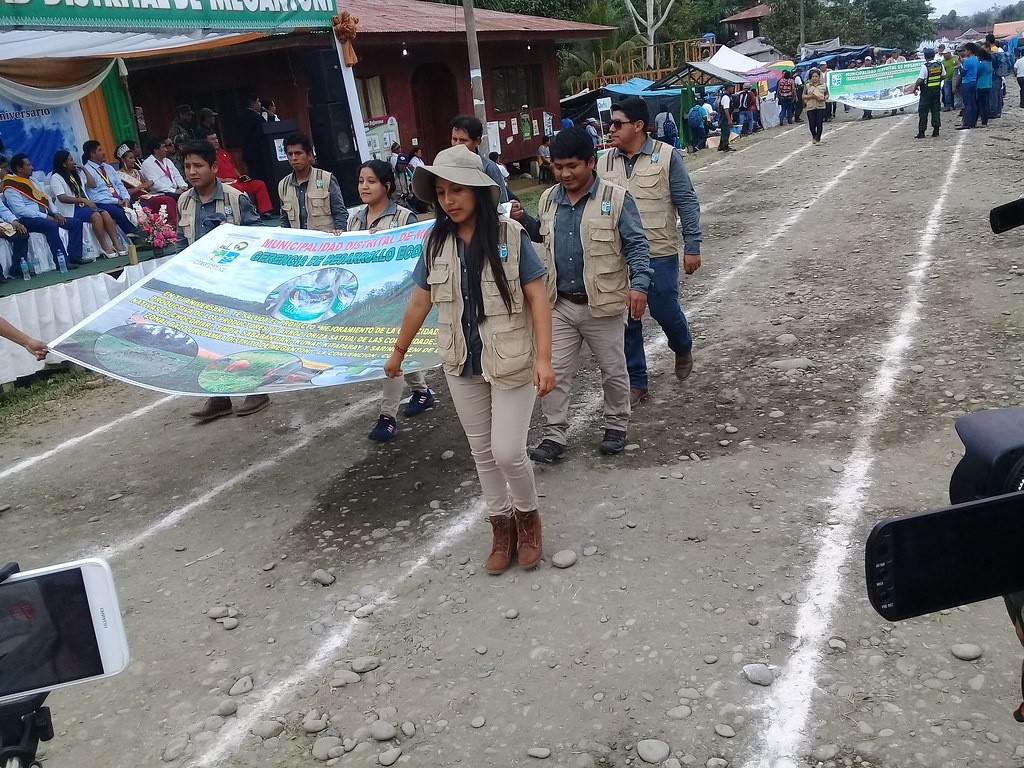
[1,253,95,283]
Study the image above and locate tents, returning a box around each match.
[797,45,897,69]
[707,44,771,73]
[561,78,724,139]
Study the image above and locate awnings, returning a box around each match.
[0,0,371,163]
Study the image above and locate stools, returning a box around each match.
[538,167,551,184]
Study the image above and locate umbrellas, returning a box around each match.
[750,60,795,92]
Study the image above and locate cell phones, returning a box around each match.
[0,559,130,706]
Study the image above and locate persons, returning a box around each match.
[509,127,654,464]
[774,61,836,146]
[350,160,435,439]
[682,81,764,152]
[1,317,49,361]
[537,136,555,183]
[385,116,556,574]
[114,98,288,252]
[596,96,701,407]
[174,138,270,420]
[820,34,1024,138]
[0,150,128,284]
[276,131,350,236]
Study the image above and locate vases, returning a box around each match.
[152,245,164,259]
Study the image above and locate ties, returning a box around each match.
[98,166,121,200]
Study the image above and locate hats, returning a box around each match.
[589,118,597,122]
[410,145,501,209]
[794,44,977,66]
[744,83,752,88]
[724,81,735,87]
[397,153,409,163]
[199,108,218,117]
[176,105,195,115]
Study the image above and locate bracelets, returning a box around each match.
[394,344,408,354]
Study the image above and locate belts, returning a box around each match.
[557,289,588,304]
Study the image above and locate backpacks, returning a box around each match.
[991,52,1011,77]
[663,94,749,138]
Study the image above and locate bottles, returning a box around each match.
[32,254,42,275]
[20,257,31,280]
[57,250,67,273]
[82,239,96,262]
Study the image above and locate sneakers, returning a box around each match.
[674,350,693,380]
[629,388,648,406]
[531,438,566,461]
[368,415,397,442]
[188,397,233,419]
[599,427,627,455]
[404,389,433,417]
[236,394,271,415]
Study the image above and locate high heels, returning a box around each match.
[111,243,129,255]
[99,244,119,259]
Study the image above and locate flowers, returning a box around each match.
[125,204,178,249]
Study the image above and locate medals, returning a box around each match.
[109,187,115,193]
[173,183,176,189]
[79,203,84,207]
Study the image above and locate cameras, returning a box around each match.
[864,406,1024,644]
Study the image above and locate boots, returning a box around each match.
[514,507,543,569]
[484,512,517,574]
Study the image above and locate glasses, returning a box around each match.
[165,142,173,147]
[608,118,632,130]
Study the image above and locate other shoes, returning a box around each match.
[718,145,736,152]
[779,106,1007,147]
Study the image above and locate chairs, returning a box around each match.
[0,170,135,280]
